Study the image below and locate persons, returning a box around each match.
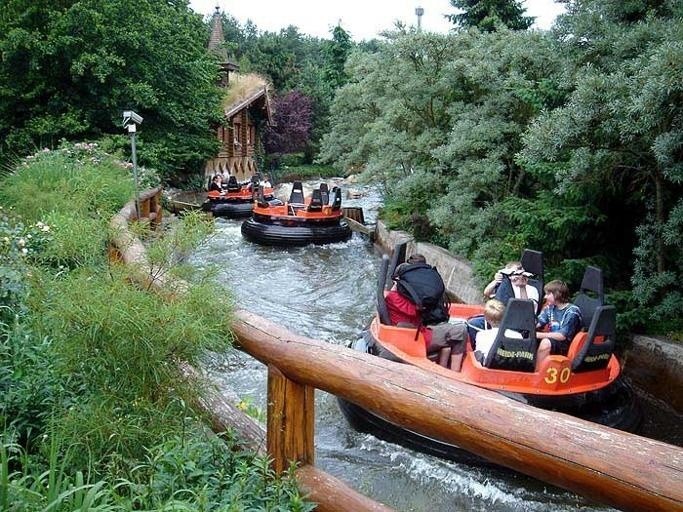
[407,254,427,266]
[474,298,523,367]
[210,175,223,192]
[482,260,539,317]
[259,177,271,187]
[532,278,584,370]
[386,262,468,372]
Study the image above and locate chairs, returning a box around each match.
[375,237,618,393]
[205,170,344,224]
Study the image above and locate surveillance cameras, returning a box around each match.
[122,110,144,125]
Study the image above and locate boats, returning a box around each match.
[337,241,644,466]
[201,172,351,246]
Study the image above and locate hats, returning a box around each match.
[495,261,534,277]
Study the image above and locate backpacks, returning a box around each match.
[391,261,451,325]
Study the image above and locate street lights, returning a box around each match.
[122,110,144,220]
